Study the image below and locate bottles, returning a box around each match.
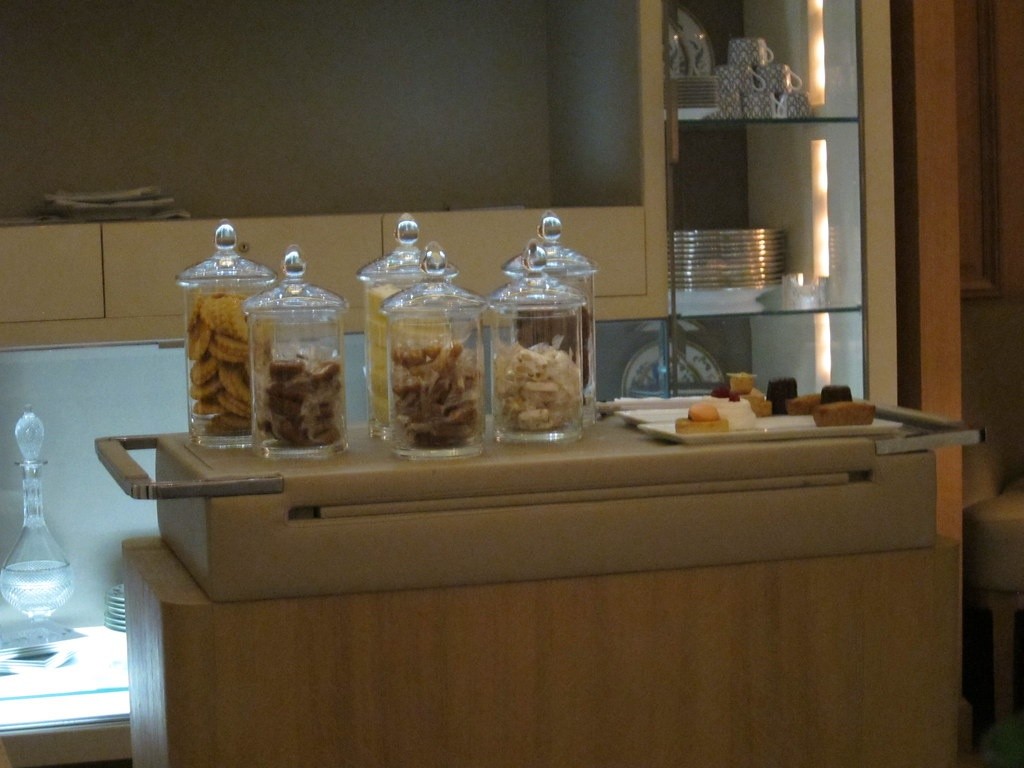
[1,404,74,620]
[177,223,598,457]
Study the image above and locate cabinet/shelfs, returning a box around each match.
[95,397,989,768]
[1,2,865,398]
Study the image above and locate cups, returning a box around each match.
[742,89,784,119]
[715,90,743,119]
[715,64,767,95]
[756,62,803,96]
[727,38,774,70]
[775,90,814,119]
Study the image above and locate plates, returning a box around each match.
[664,75,720,120]
[607,396,729,413]
[613,393,822,425]
[104,582,127,632]
[671,226,789,288]
[636,413,902,444]
